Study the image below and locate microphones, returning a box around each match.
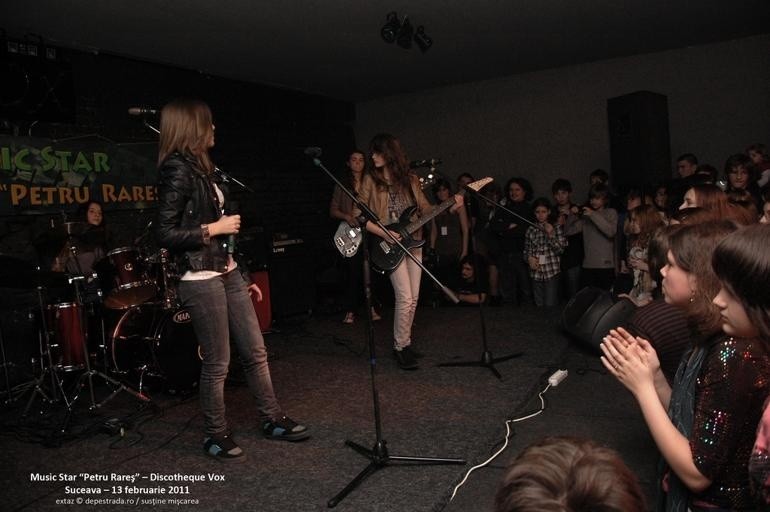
[408,157,444,168]
[226,198,240,254]
[129,106,157,118]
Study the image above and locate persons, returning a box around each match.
[598,220,770,510]
[709,221,769,341]
[420,146,769,377]
[330,146,385,326]
[493,432,649,512]
[746,390,770,511]
[148,91,315,465]
[353,132,467,373]
[36,199,107,306]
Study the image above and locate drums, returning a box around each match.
[111,301,204,401]
[59,239,107,280]
[149,248,184,312]
[46,301,88,372]
[92,247,160,310]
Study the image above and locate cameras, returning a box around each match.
[573,206,585,217]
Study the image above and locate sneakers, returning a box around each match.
[343,311,354,324]
[396,349,420,369]
[261,414,310,441]
[371,307,381,321]
[392,344,425,360]
[202,430,248,464]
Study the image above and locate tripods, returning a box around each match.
[0,337,36,406]
[58,276,151,436]
[326,218,468,508]
[436,189,525,380]
[21,284,78,419]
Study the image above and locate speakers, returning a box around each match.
[607,89,670,193]
[561,283,636,351]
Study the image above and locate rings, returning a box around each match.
[614,364,620,370]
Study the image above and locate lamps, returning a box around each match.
[380,9,434,53]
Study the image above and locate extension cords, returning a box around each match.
[547,366,568,388]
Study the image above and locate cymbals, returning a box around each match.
[50,222,101,236]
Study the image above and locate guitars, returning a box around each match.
[329,174,437,258]
[367,177,493,274]
